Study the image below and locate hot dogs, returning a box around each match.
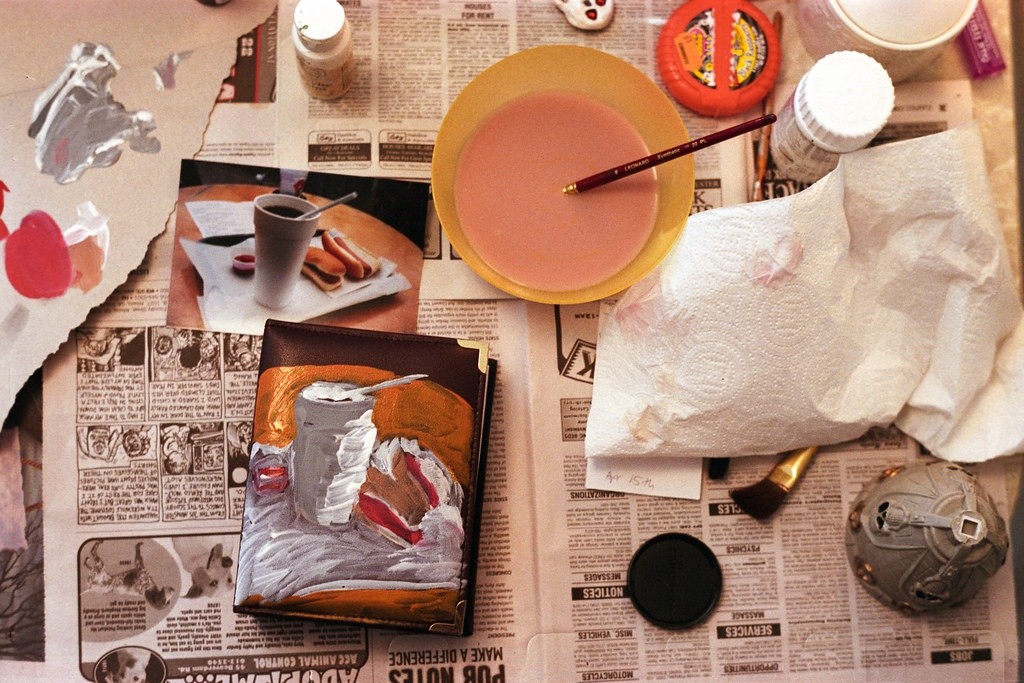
[304,229,381,290]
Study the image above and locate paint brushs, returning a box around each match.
[708,457,730,481]
[728,444,820,521]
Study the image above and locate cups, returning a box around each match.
[253,193,321,310]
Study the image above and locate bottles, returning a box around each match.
[770,50,896,184]
[291,0,356,101]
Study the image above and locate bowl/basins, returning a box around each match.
[430,43,696,305]
[232,253,255,281]
